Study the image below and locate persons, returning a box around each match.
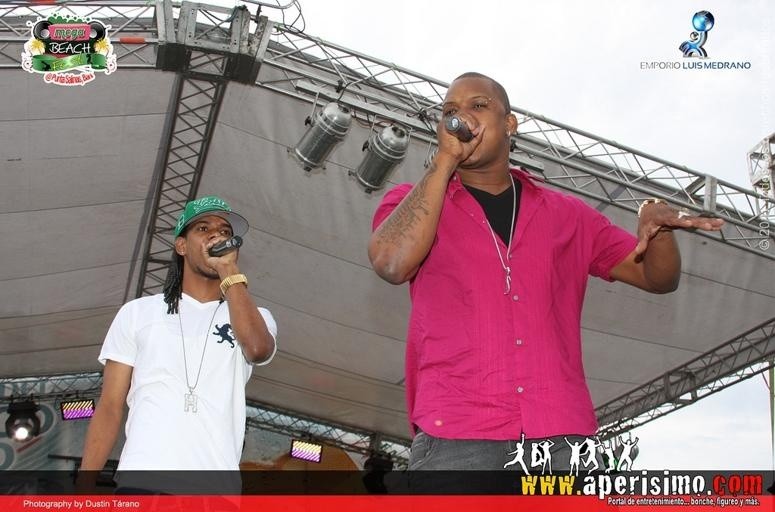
[75,196,277,496]
[368,73,724,495]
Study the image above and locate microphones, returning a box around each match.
[445,115,474,142]
[209,235,243,257]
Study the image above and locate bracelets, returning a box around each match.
[219,273,248,296]
[638,197,667,220]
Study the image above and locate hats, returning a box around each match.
[174,196,249,238]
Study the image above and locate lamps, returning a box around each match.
[287,79,414,197]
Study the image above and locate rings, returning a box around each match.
[678,207,691,222]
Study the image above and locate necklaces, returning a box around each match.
[176,296,222,412]
[485,172,520,290]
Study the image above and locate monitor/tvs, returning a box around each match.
[291,439,322,463]
[60,399,95,420]
